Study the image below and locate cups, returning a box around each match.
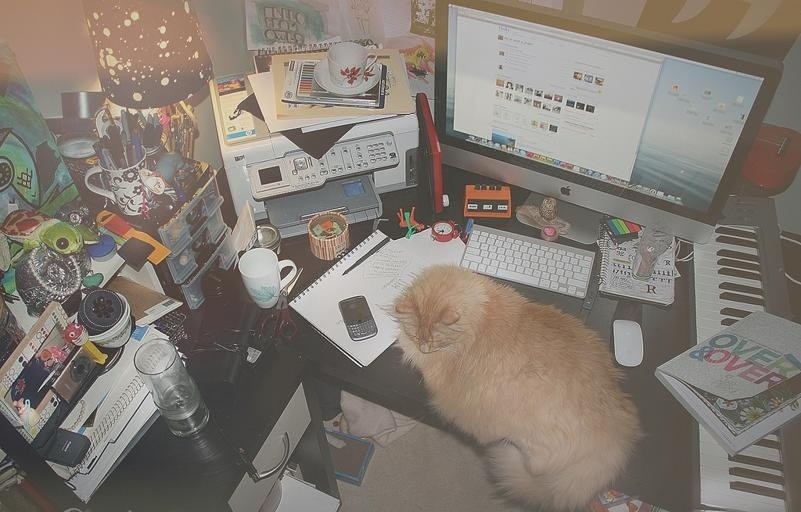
[150,370,210,439]
[307,210,350,260]
[82,146,152,216]
[79,295,130,347]
[145,140,163,172]
[236,247,298,312]
[630,218,676,282]
[133,338,199,421]
[58,135,106,199]
[326,42,377,89]
[251,224,281,256]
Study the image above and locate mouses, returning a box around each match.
[612,319,644,369]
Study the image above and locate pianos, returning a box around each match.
[695,195,801,512]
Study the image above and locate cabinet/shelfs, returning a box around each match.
[0,299,341,512]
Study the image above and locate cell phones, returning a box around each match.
[338,295,378,341]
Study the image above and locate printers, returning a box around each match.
[208,45,422,237]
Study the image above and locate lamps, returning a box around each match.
[81,0,214,110]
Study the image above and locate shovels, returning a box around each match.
[95,209,173,267]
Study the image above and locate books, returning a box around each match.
[598,220,676,306]
[282,56,384,107]
[286,226,425,370]
[653,309,801,458]
[255,49,417,133]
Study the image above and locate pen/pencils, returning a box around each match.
[342,238,389,276]
[92,109,145,170]
[138,111,161,148]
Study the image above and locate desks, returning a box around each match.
[173,148,800,510]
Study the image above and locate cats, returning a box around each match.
[379,265,642,512]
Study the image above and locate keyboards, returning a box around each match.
[459,223,596,300]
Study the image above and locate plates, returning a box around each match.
[313,56,382,96]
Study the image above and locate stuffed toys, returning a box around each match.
[20,214,101,258]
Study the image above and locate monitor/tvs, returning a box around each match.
[435,0,784,246]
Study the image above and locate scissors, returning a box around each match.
[260,288,298,342]
